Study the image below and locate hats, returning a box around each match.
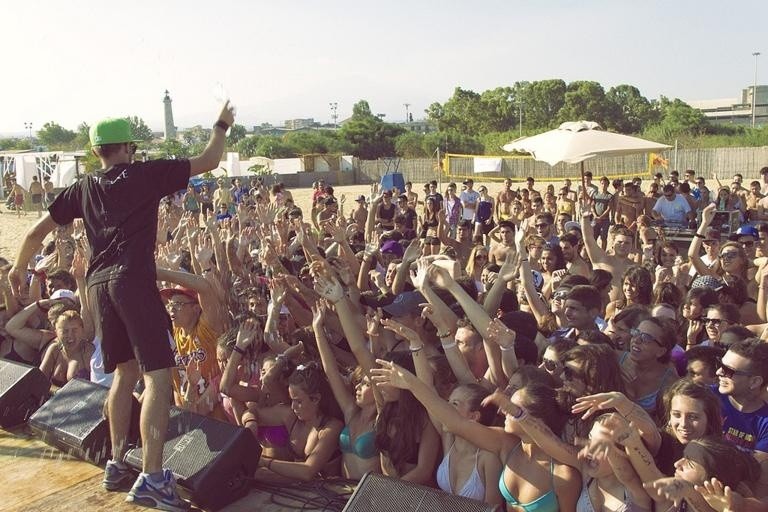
[701,229,720,241]
[355,190,408,202]
[691,275,723,291]
[160,284,198,301]
[88,119,144,145]
[382,291,427,317]
[381,241,404,257]
[734,226,759,238]
[51,289,77,303]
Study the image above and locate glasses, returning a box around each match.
[740,240,754,247]
[535,222,549,229]
[544,358,573,381]
[165,301,197,310]
[702,319,754,378]
[630,328,664,347]
[719,251,737,260]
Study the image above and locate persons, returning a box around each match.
[7,99,236,511]
[0,171,768,511]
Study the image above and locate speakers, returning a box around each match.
[26,374,142,466]
[339,471,501,512]
[0,355,52,429]
[121,404,263,512]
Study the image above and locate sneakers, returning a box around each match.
[102,460,136,492]
[125,469,191,512]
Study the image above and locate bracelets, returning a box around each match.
[212,120,230,132]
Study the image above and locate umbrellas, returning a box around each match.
[498,117,675,193]
